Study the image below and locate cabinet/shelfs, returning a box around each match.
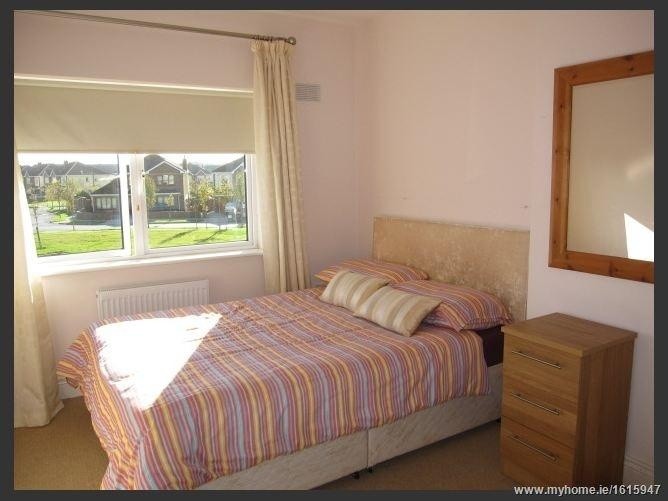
[497,311,638,494]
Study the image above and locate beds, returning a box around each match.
[67,217,530,490]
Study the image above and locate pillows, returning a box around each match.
[313,256,513,338]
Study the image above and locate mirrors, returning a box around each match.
[547,48,656,285]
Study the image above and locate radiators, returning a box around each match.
[97,278,211,320]
